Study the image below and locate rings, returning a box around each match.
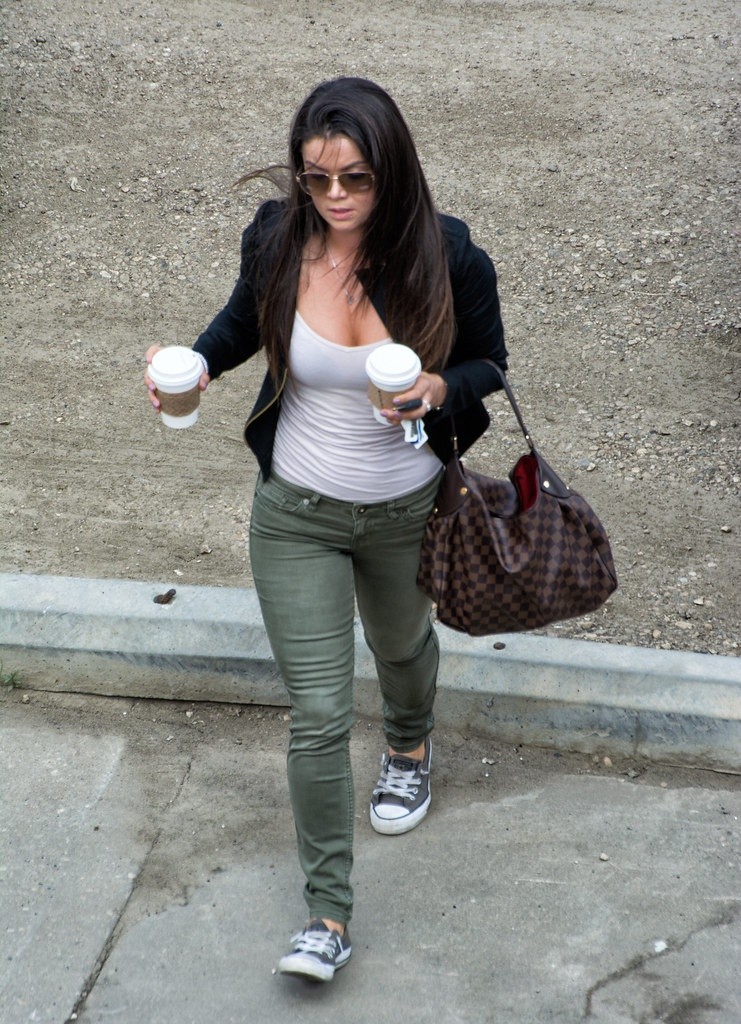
[422,398,433,413]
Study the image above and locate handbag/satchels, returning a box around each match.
[417,357,618,636]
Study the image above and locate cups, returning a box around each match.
[365,344,422,426]
[147,346,203,429]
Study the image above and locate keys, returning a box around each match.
[392,398,422,413]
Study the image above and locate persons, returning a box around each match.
[142,79,373,438]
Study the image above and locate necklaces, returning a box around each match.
[322,229,366,304]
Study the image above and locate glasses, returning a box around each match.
[295,160,378,195]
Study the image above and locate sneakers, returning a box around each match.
[278,918,350,981]
[370,736,432,835]
